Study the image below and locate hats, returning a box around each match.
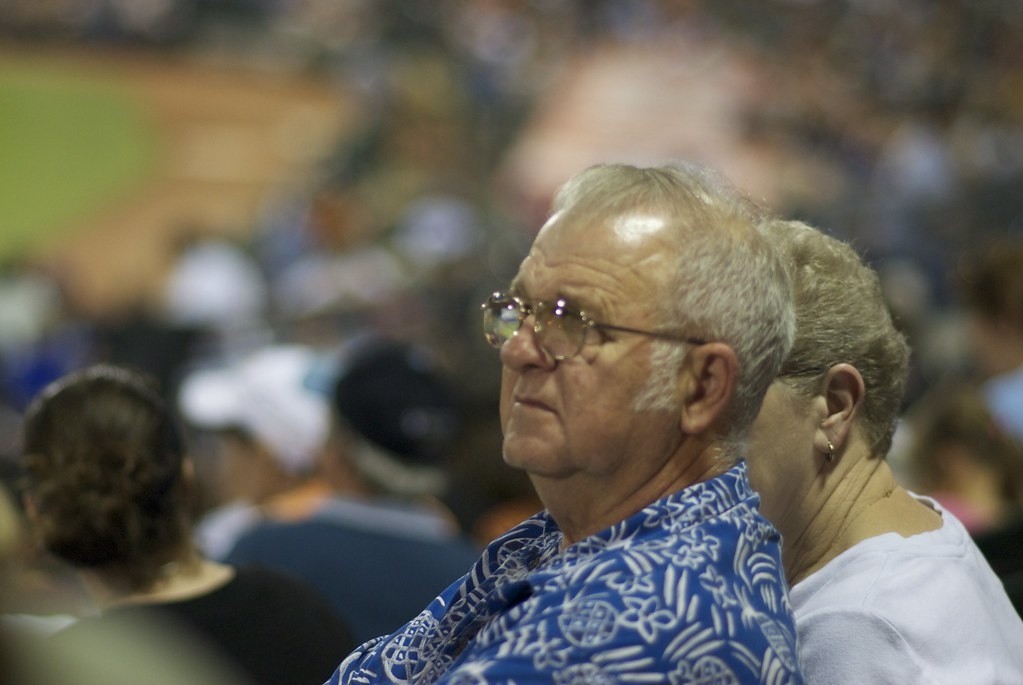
[303,338,464,463]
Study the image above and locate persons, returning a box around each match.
[0,192,1023,685]
[745,222,1023,685]
[321,162,801,684]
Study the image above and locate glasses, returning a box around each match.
[482,289,706,362]
